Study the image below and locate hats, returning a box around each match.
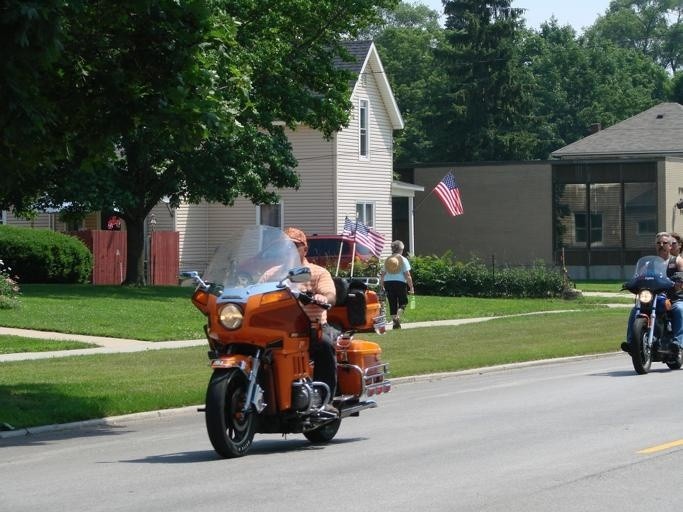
[280,227,307,247]
[385,254,404,274]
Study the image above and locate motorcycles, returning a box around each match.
[621,256,683,375]
[177,224,391,458]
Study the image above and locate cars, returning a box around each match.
[223,233,383,289]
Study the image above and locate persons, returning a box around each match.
[258,225,340,415]
[668,232,683,258]
[380,239,415,329]
[619,231,683,351]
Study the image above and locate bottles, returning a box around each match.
[410,292,415,310]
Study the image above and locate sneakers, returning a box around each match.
[392,316,402,329]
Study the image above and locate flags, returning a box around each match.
[432,172,464,217]
[355,218,385,258]
[342,216,373,242]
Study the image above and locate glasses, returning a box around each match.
[656,241,667,245]
[672,242,677,245]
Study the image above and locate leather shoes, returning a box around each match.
[324,404,339,413]
[621,342,632,356]
